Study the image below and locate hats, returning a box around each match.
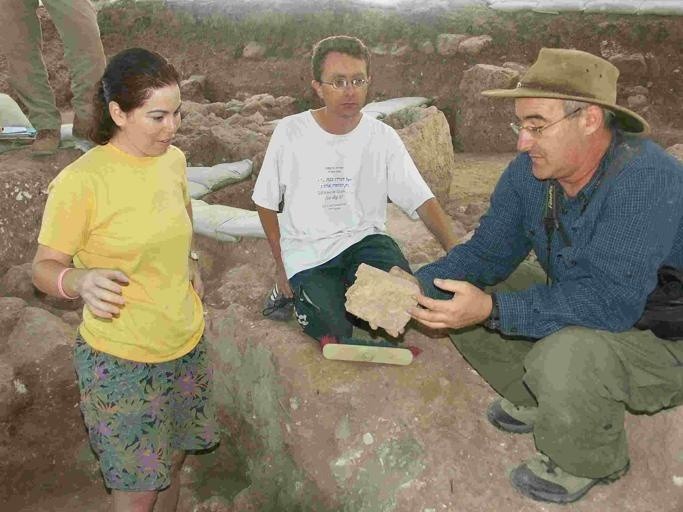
[481,46,650,135]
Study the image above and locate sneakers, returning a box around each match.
[487,398,538,433]
[509,450,630,505]
[265,281,294,322]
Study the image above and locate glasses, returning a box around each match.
[318,77,369,90]
[510,108,580,135]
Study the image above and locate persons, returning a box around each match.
[368,47,683,504]
[0,0,107,156]
[251,35,460,341]
[31,48,220,512]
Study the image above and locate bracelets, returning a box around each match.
[57,267,80,300]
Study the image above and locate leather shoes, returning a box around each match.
[31,129,60,155]
[71,113,97,139]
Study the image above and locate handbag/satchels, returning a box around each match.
[635,266,683,341]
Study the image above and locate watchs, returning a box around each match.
[189,250,200,262]
[483,292,502,330]
